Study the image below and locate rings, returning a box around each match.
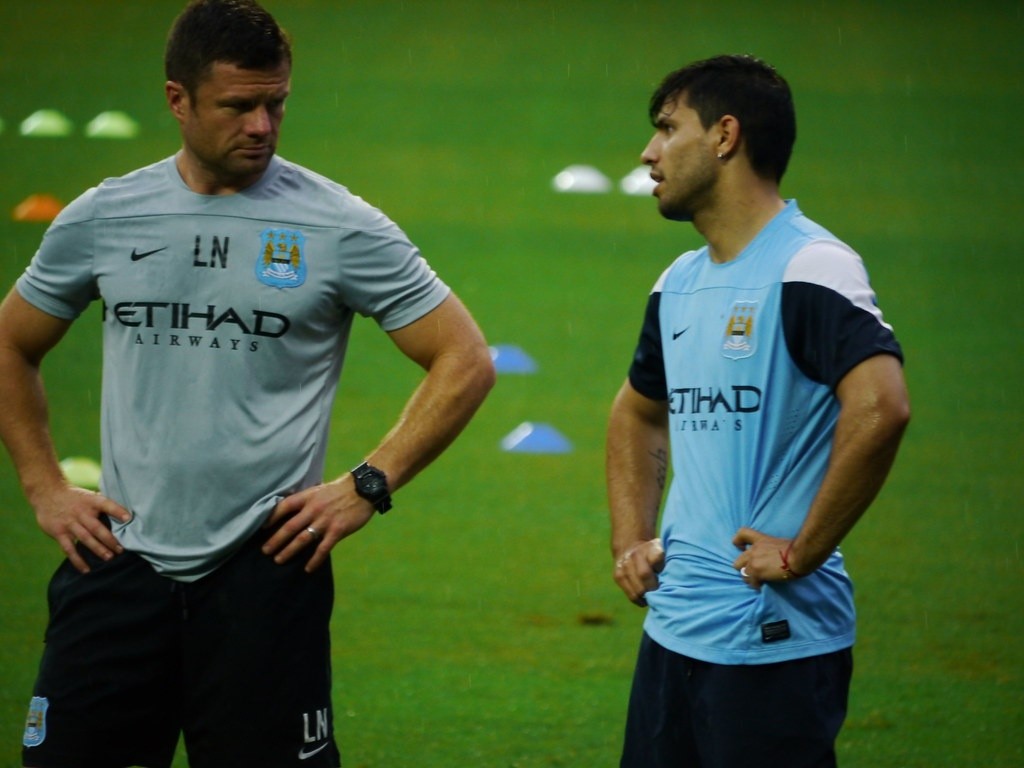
[308,528,317,538]
[741,567,749,579]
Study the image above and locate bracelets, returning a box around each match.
[780,540,795,578]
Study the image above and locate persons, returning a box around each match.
[1,2,496,767]
[605,55,912,767]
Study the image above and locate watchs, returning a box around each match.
[351,461,394,516]
